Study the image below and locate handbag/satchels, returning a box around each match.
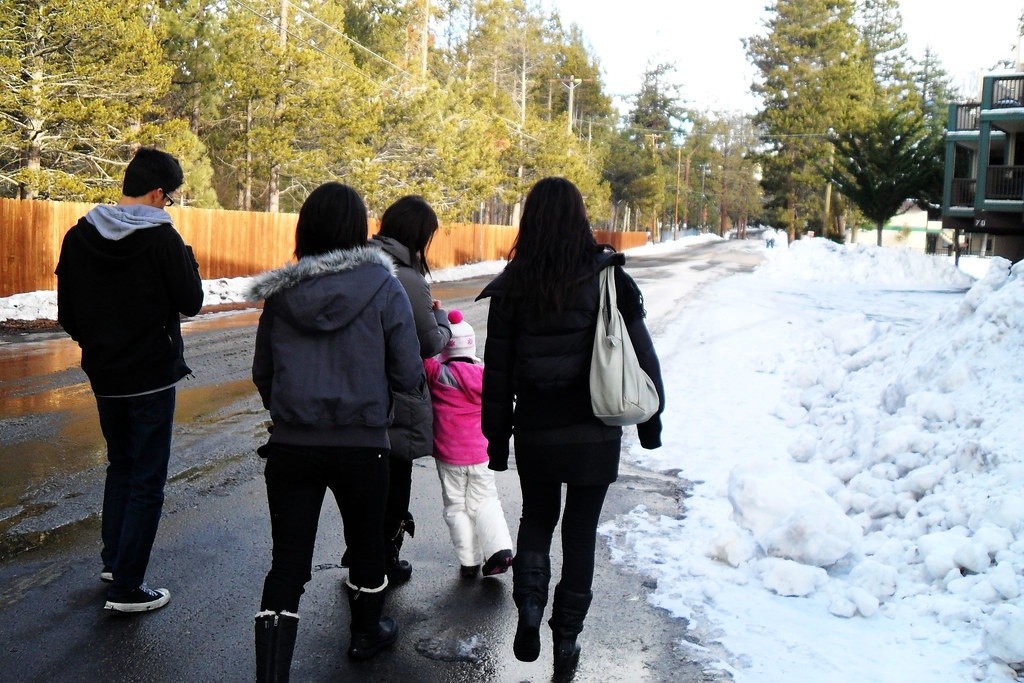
[589,247,660,426]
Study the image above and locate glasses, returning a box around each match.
[166,193,174,206]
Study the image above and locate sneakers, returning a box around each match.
[104,582,171,612]
[100,567,113,580]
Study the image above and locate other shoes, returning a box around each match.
[482,550,513,576]
[460,565,481,578]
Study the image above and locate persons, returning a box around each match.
[338,192,452,587]
[474,176,665,683]
[252,179,425,683]
[57,146,205,613]
[422,310,514,578]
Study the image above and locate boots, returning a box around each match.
[548,583,592,683]
[341,549,350,567]
[389,511,412,588]
[345,575,399,658]
[512,553,551,662]
[254,609,300,683]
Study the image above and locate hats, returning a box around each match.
[437,310,481,365]
